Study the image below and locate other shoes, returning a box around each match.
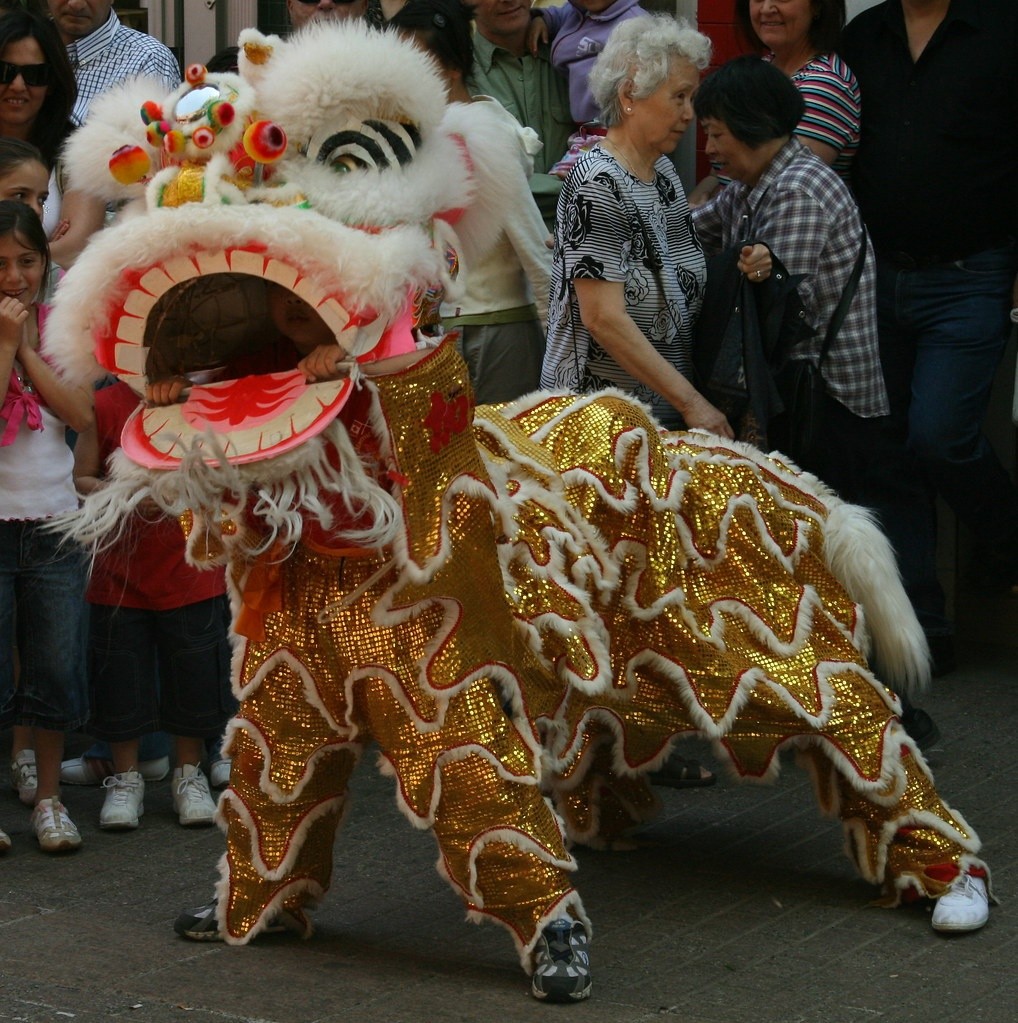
[972,543,1017,594]
[927,632,980,671]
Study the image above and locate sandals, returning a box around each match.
[654,752,716,788]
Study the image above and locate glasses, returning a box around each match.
[0,61,52,86]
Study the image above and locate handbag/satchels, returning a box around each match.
[759,356,825,463]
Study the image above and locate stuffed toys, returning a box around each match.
[47,17,991,965]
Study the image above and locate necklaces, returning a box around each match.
[606,134,641,180]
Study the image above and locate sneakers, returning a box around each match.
[9,750,39,804]
[930,874,990,934]
[170,764,217,827]
[101,765,147,829]
[59,752,170,785]
[29,795,83,852]
[899,706,937,749]
[529,918,590,1002]
[175,898,289,939]
[211,759,231,786]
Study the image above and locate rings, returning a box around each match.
[757,270,761,278]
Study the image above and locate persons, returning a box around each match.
[0,0,1018,1002]
